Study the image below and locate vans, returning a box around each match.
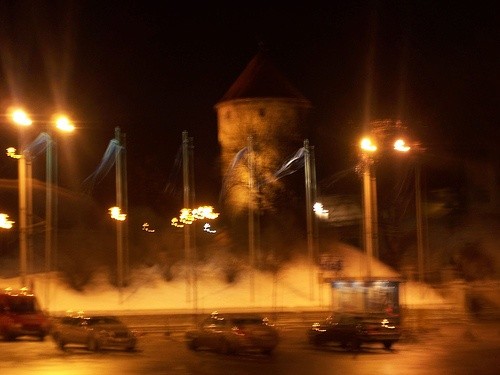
[0,287,48,341]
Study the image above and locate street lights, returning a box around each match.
[43,109,73,272]
[7,108,36,285]
[394,137,425,281]
[358,134,378,272]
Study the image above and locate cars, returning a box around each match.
[188,316,278,357]
[52,314,136,352]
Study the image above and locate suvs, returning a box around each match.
[308,311,401,349]
